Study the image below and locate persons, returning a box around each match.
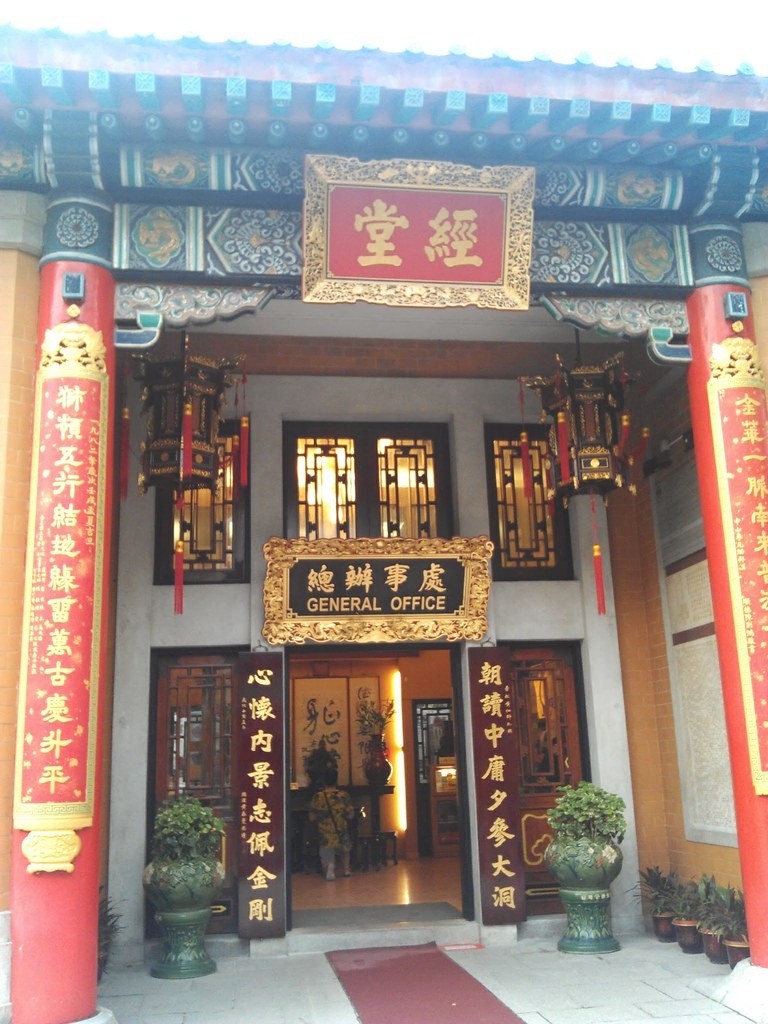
[308,767,356,882]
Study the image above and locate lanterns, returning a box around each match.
[121,326,249,615]
[517,351,646,615]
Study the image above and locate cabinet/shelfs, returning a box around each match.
[427,764,458,858]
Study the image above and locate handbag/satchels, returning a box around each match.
[341,833,352,852]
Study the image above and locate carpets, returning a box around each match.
[291,901,464,931]
[323,939,526,1024]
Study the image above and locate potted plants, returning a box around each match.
[624,865,752,972]
[141,796,227,979]
[544,780,628,954]
[354,700,396,786]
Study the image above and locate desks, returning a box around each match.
[290,786,395,874]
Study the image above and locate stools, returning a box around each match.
[377,831,398,867]
[356,834,381,872]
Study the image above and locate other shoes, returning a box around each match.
[344,867,353,876]
[326,863,336,881]
